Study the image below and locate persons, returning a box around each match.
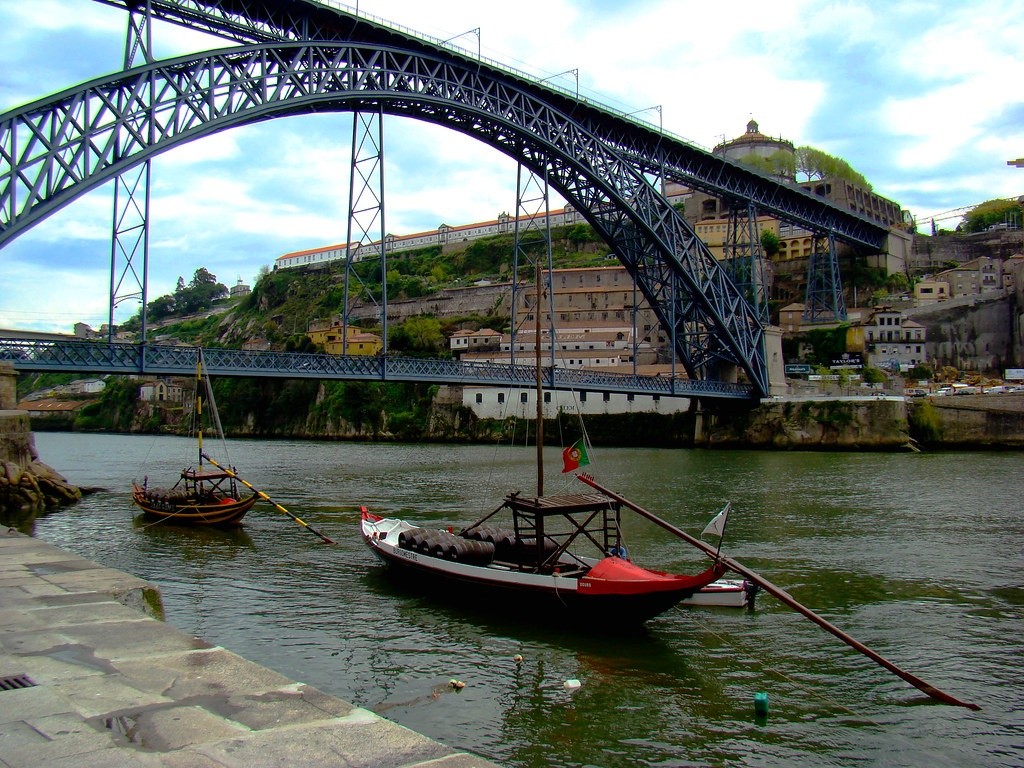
[609,539,627,561]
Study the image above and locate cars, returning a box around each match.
[903,379,1024,399]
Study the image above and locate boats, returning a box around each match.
[132,347,260,527]
[359,261,730,625]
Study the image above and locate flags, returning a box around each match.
[701,501,731,537]
[561,437,591,474]
